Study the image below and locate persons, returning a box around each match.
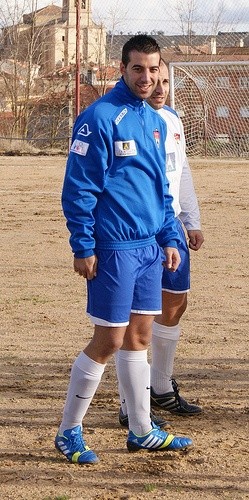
[54,34,191,463]
[115,57,205,427]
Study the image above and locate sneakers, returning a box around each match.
[150,378,203,415]
[127,422,192,451]
[55,425,99,464]
[119,408,167,427]
